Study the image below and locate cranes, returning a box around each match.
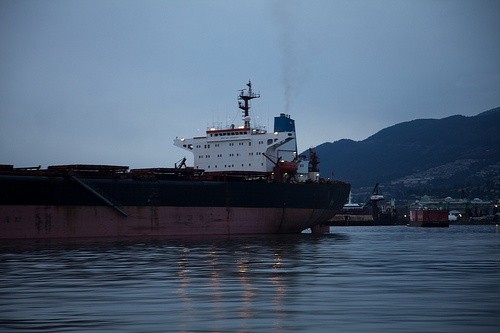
[363,182,386,226]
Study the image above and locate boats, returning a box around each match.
[448,211,462,221]
[407,208,450,228]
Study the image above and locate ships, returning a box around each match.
[0,79,350,247]
